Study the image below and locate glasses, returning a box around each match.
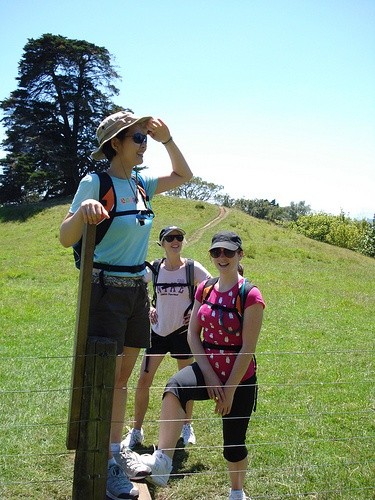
[210,248,236,258]
[123,132,147,144]
[164,235,183,242]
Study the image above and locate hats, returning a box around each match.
[89,111,153,160]
[160,225,186,245]
[208,231,243,251]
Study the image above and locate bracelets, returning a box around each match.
[165,140,173,147]
[162,136,172,144]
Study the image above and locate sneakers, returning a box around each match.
[229,491,247,500]
[114,445,152,479]
[121,426,145,448]
[182,425,196,445]
[105,459,140,500]
[140,444,173,487]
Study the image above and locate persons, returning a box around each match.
[59,110,192,500]
[133,230,265,500]
[122,225,213,449]
